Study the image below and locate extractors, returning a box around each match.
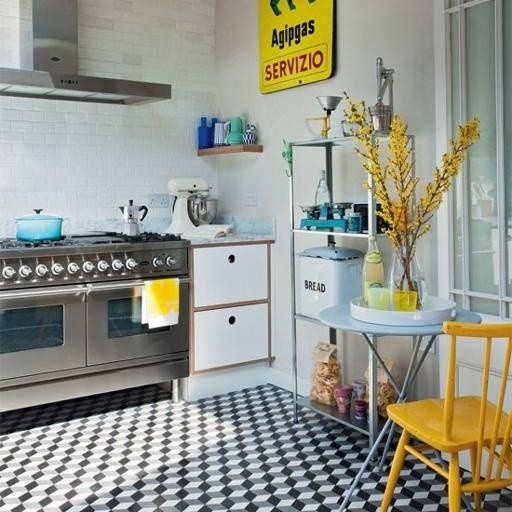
[1,0,171,104]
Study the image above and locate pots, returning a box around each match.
[12,208,64,243]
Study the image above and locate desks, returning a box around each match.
[318,301,481,512]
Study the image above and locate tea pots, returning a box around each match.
[119,199,148,237]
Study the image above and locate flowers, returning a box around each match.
[339,91,483,308]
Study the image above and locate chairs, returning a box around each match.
[379,321,512,512]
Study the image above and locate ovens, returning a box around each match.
[0,276,190,415]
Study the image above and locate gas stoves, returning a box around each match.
[0,231,190,290]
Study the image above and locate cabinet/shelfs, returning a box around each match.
[157,244,277,404]
[287,133,416,463]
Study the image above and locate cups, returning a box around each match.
[366,288,418,312]
[332,379,367,420]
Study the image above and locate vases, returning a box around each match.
[389,231,427,312]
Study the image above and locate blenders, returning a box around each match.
[166,176,218,236]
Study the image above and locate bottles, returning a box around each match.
[198,117,257,150]
[347,212,362,233]
[362,234,384,304]
